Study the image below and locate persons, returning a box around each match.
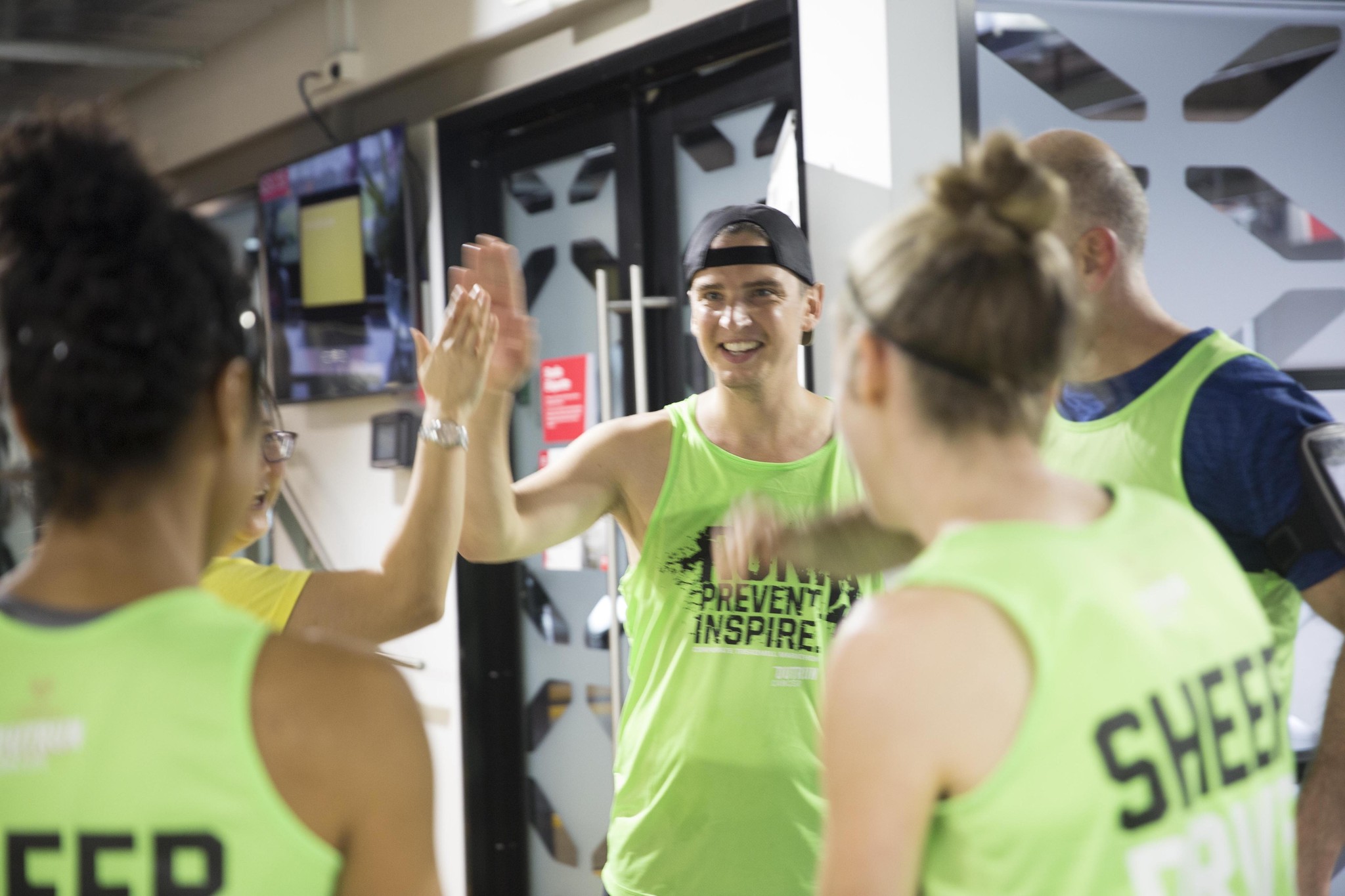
[710,128,1345,896]
[0,110,500,896]
[447,203,893,896]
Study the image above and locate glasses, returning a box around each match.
[260,430,297,465]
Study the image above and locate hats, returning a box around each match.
[685,205,814,286]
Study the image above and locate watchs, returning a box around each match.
[415,418,466,449]
